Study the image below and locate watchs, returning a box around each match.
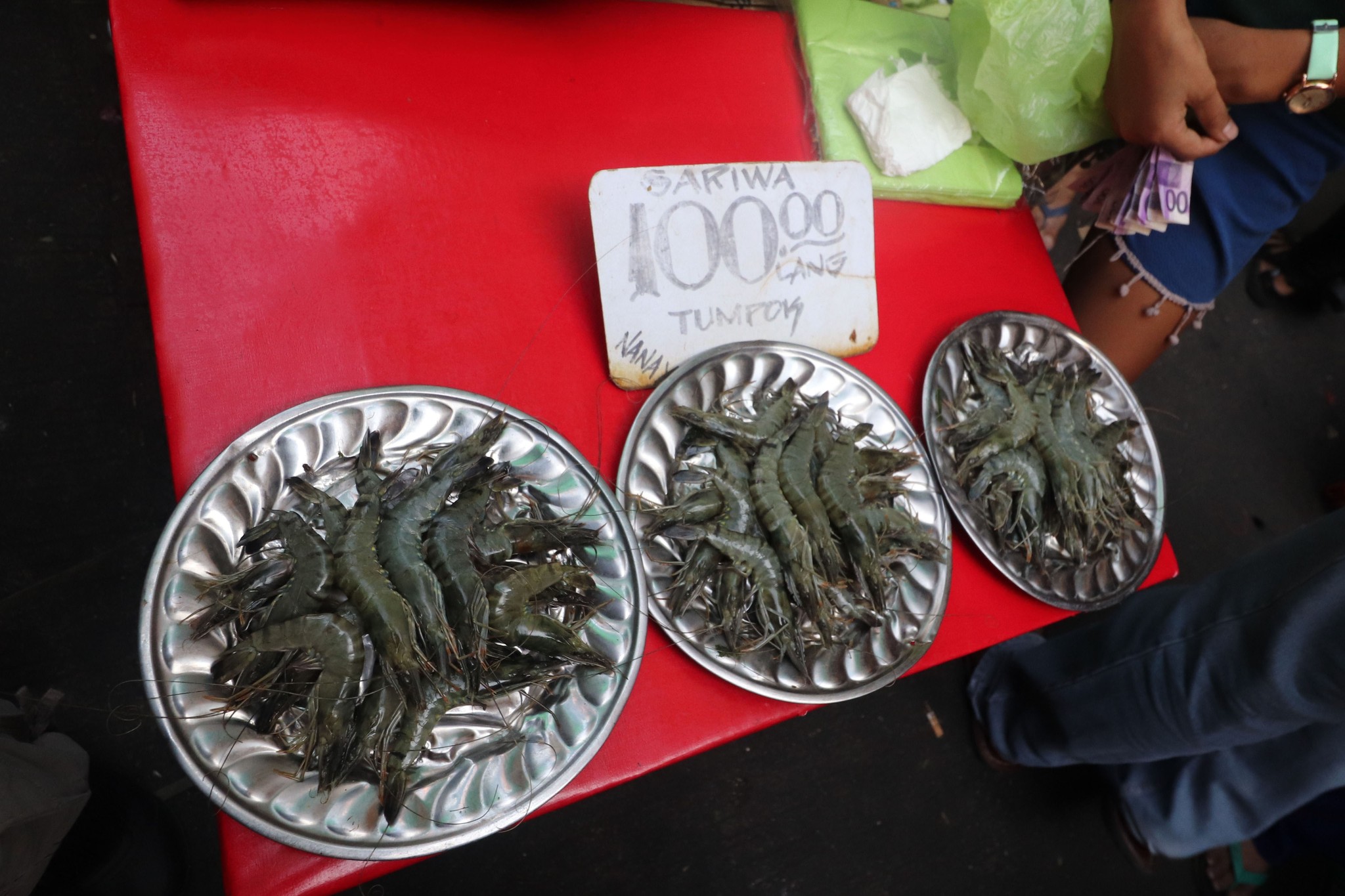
[1285,17,1338,113]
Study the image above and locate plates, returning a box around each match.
[136,385,649,861]
[615,340,953,703]
[922,309,1167,612]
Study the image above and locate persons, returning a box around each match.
[962,507,1345,865]
[1205,786,1345,896]
[1025,0,1345,388]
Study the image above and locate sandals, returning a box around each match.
[1247,234,1312,306]
[1196,838,1271,896]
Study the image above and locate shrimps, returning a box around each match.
[179,407,620,825]
[935,347,1143,564]
[633,375,951,683]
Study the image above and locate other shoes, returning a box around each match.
[968,631,1040,769]
[1105,764,1159,872]
